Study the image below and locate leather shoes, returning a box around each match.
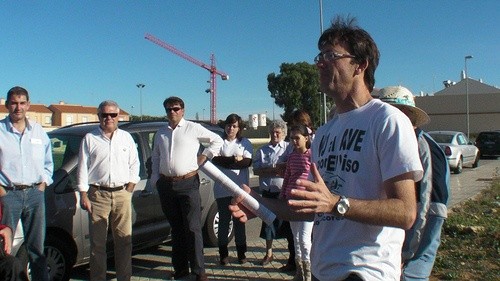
[168,268,189,279]
[196,273,207,281]
[261,254,274,265]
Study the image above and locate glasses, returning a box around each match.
[313,51,356,62]
[166,107,183,111]
[288,136,301,141]
[98,113,119,118]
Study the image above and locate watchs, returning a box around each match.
[333,195,350,219]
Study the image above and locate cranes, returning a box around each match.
[143,33,229,124]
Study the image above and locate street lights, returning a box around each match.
[136,83,149,123]
[464,56,474,139]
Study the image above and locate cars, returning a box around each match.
[476,131,500,158]
[12,121,234,281]
[427,131,480,173]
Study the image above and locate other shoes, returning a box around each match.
[221,256,233,265]
[238,257,248,263]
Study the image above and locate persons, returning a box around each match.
[0,86,54,280]
[275,110,316,176]
[148,96,225,281]
[370,85,455,281]
[251,118,294,267]
[80,101,144,281]
[284,14,425,281]
[280,123,315,281]
[212,114,253,266]
[0,200,30,280]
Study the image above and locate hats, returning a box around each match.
[377,86,430,127]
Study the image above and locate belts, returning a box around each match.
[89,184,128,192]
[159,170,198,181]
[4,183,40,190]
